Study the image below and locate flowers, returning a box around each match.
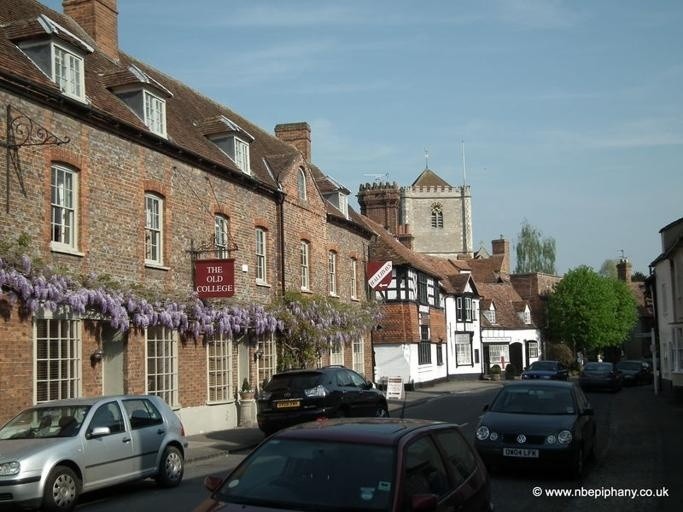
[0,236,384,353]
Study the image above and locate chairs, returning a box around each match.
[133,410,147,425]
[59,416,78,436]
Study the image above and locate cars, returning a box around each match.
[578,357,661,392]
[470,381,602,476]
[521,360,570,383]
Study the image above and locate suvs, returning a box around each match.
[256,363,391,440]
[186,417,500,512]
[0,392,187,510]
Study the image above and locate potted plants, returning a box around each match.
[239,378,255,399]
[489,365,501,380]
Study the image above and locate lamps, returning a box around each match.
[90,350,104,362]
[254,350,264,363]
